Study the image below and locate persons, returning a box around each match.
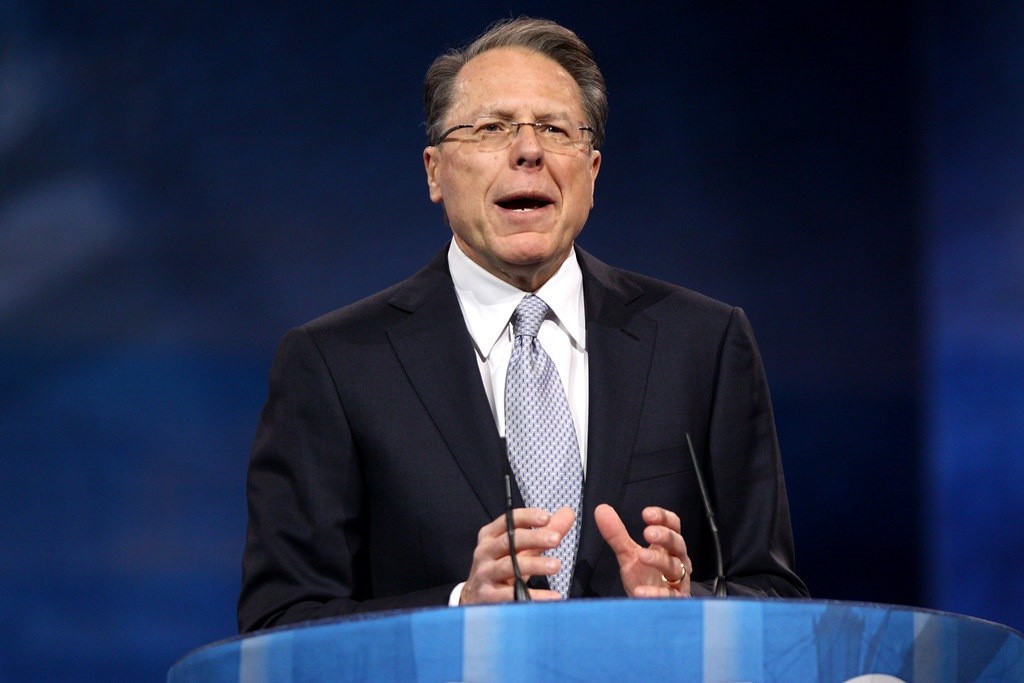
[237,17,808,633]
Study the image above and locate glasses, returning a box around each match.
[435,118,599,156]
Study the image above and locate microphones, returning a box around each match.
[678,408,729,598]
[497,437,531,602]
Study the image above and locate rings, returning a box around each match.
[662,564,686,585]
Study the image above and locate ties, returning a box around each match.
[505,294,585,599]
